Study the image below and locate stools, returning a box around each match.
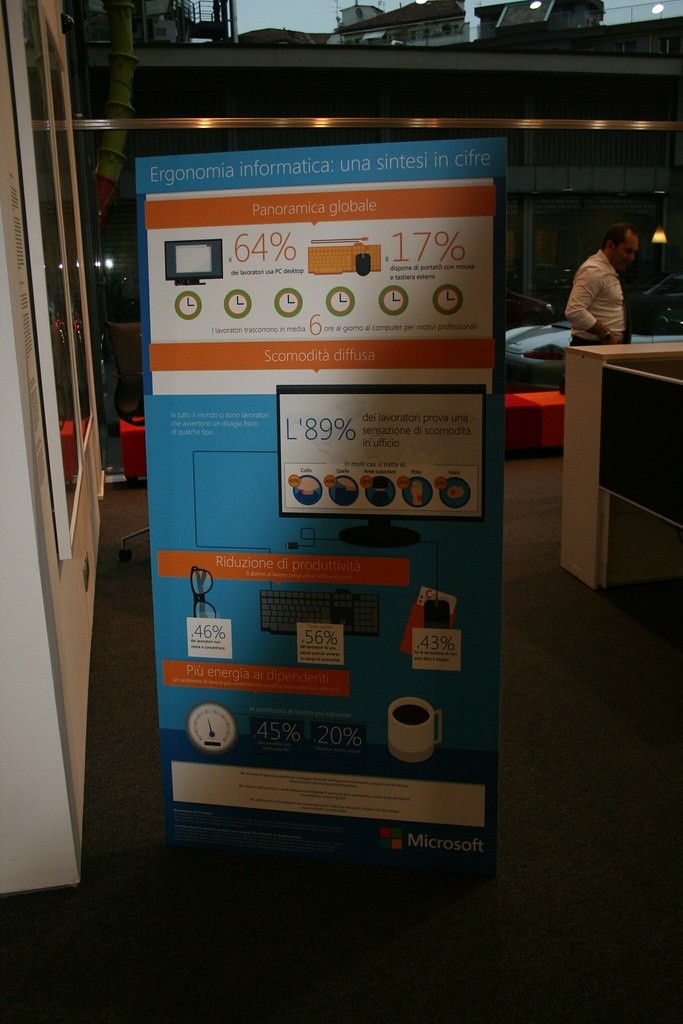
[118,414,147,487]
[59,417,75,485]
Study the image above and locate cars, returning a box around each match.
[505,289,556,331]
[621,273,683,299]
[506,293,683,392]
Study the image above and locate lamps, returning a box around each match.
[650,197,670,245]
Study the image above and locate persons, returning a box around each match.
[565,222,639,346]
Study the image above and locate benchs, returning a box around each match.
[502,388,566,449]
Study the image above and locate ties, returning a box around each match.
[618,271,631,343]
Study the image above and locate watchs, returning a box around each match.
[601,328,610,340]
